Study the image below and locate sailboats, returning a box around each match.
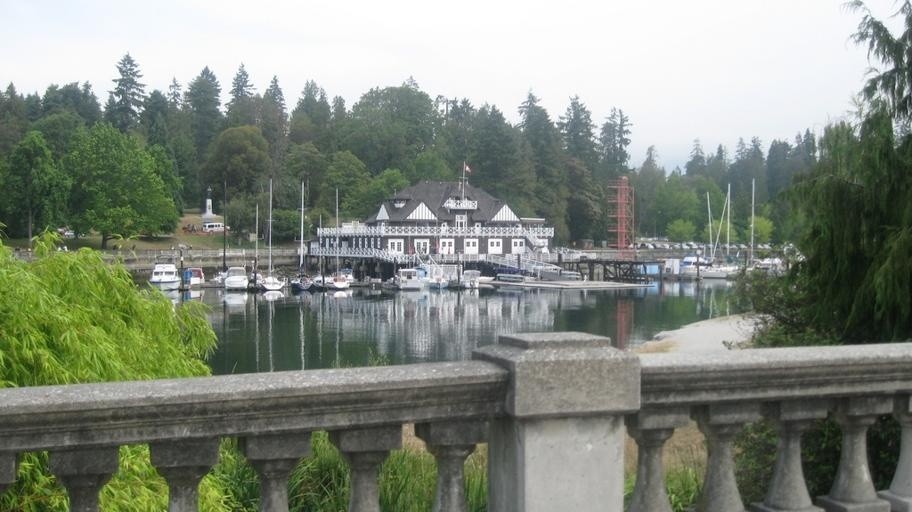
[694,275,756,321]
[621,179,806,281]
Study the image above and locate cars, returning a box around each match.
[64,230,85,236]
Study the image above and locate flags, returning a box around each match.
[465,164,472,174]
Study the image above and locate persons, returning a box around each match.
[184,268,192,284]
